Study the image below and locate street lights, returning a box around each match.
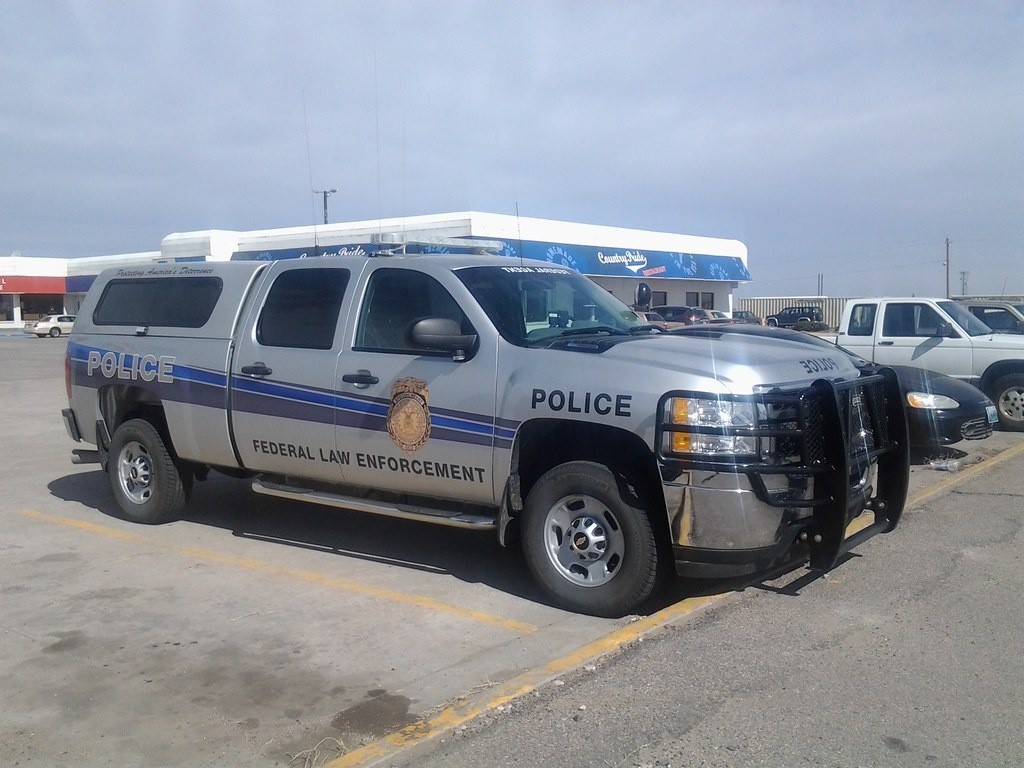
[313,188,340,225]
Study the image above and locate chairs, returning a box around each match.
[385,295,428,318]
[888,320,901,334]
[461,288,497,335]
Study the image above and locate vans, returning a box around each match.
[948,293,1024,334]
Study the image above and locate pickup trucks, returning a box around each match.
[60,233,912,619]
[806,298,1024,433]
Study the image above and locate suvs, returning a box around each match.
[766,306,823,328]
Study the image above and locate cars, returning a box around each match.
[733,311,762,326]
[22,314,77,338]
[668,321,999,452]
[634,306,731,330]
